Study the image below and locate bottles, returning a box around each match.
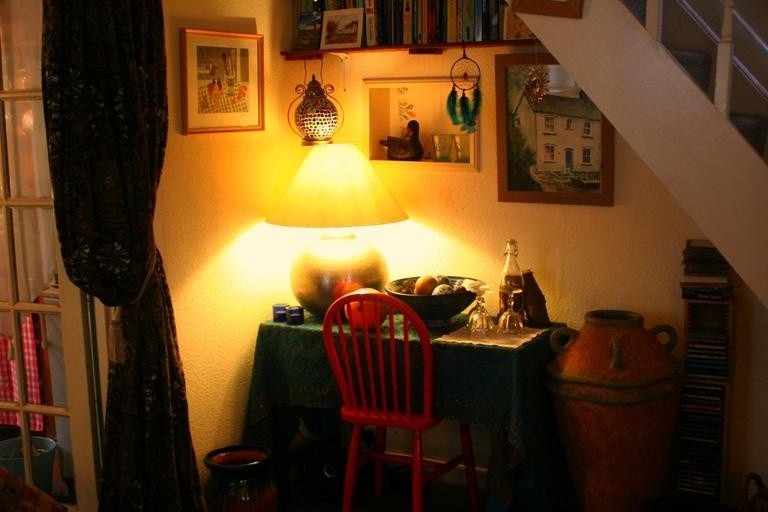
[497,239,526,326]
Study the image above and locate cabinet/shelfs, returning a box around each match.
[668,296,737,510]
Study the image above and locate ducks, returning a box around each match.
[379,119,423,161]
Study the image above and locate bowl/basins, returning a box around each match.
[385,274,483,329]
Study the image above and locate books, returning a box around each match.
[292,0,536,49]
[675,239,730,497]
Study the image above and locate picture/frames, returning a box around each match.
[178,27,265,135]
[494,53,615,208]
[320,8,364,50]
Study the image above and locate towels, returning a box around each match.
[1,295,56,439]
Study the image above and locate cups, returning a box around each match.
[431,134,471,163]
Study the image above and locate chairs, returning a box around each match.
[322,292,481,512]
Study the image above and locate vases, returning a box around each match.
[544,309,685,512]
[202,444,279,512]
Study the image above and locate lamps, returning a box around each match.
[287,57,345,147]
[264,142,410,315]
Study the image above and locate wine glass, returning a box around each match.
[464,279,496,336]
[498,285,524,337]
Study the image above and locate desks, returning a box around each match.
[242,310,570,512]
